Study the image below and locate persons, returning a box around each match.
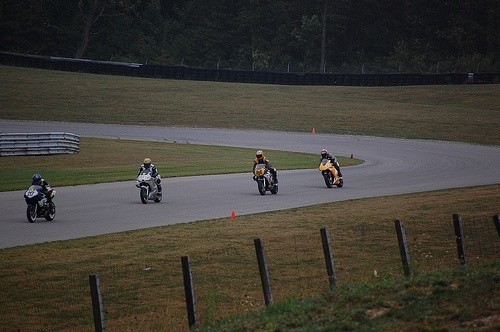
[137,158,162,195]
[253,150,278,185]
[319,149,343,179]
[25,174,55,215]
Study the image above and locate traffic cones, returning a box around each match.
[311,128,316,136]
[231,211,236,221]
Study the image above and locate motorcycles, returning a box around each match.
[23,185,58,223]
[136,170,164,204]
[252,164,279,197]
[318,158,344,188]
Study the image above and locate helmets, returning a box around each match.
[256,150,264,158]
[321,149,327,155]
[143,158,152,164]
[32,174,41,182]
